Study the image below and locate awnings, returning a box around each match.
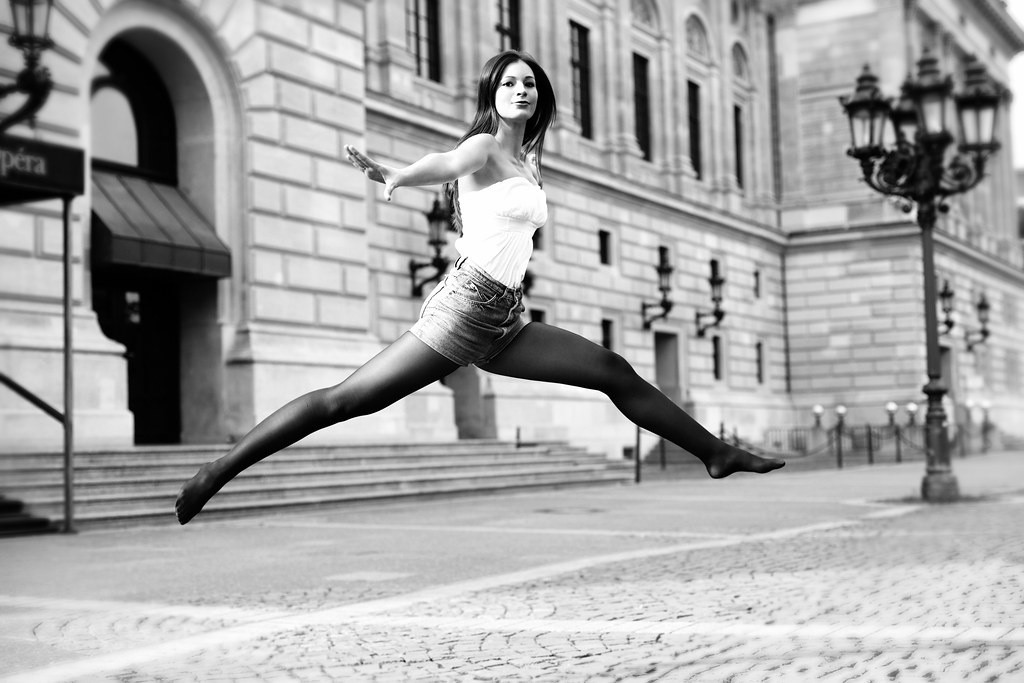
[90,171,233,278]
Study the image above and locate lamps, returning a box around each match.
[0,0,56,132]
[938,280,956,337]
[640,255,675,331]
[964,292,990,352]
[407,197,452,298]
[696,269,728,338]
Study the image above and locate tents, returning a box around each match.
[0,130,85,527]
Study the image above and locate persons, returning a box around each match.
[175,50,789,525]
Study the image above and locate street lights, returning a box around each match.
[837,44,1003,502]
